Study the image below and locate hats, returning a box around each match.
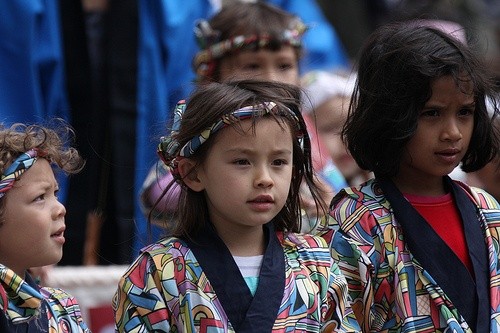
[297,69,357,116]
